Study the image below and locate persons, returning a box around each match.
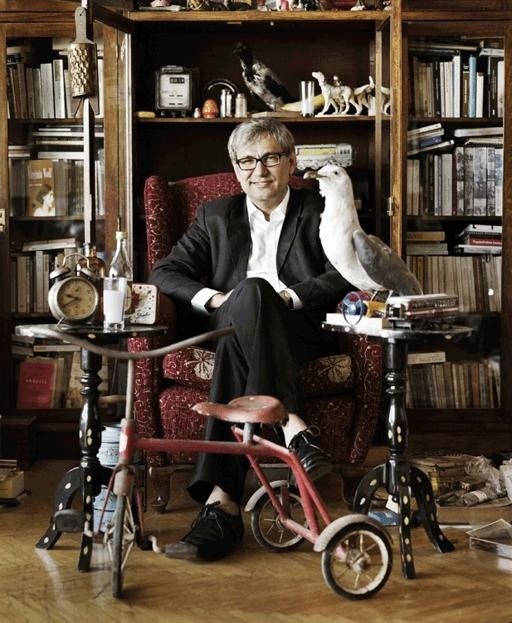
[147,115,358,562]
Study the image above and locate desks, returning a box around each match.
[14,320,170,574]
[316,317,475,582]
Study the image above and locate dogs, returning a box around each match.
[311,72,374,115]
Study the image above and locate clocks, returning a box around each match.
[46,252,102,329]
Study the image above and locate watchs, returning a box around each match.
[284,290,293,309]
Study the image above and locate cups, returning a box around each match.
[102,277,127,331]
[300,80,315,118]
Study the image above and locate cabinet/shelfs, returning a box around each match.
[1,0,512,460]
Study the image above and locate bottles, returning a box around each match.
[234,93,248,119]
[220,89,232,118]
[76,242,107,326]
[109,231,134,329]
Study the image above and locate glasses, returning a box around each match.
[234,152,288,171]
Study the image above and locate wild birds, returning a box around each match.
[302,164,424,305]
[232,42,299,111]
[352,227,423,308]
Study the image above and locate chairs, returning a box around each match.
[123,168,389,512]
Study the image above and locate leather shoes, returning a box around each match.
[165,502,245,561]
[287,430,336,488]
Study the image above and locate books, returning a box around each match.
[405,223,503,313]
[8,124,106,217]
[405,351,501,409]
[10,236,106,313]
[7,36,105,119]
[408,33,504,118]
[12,321,110,409]
[406,123,504,216]
